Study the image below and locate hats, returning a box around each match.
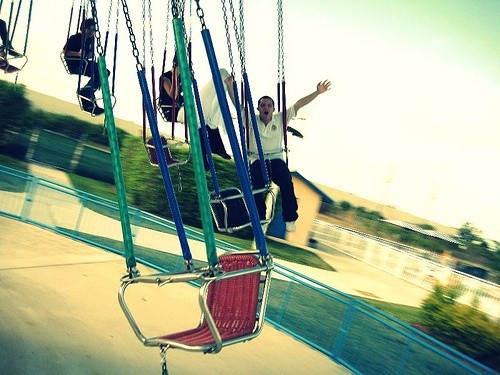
[81,18,96,27]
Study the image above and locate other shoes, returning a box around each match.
[286,221,296,232]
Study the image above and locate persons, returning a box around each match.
[224,76,330,233]
[63,18,111,109]
[0,18,20,72]
[159,55,232,172]
